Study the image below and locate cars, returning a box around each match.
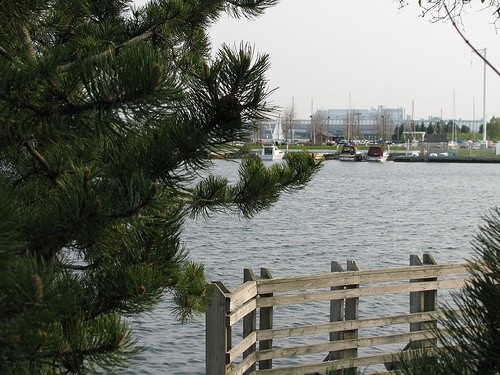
[448,140,497,150]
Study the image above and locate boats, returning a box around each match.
[339,142,361,162]
[257,144,286,160]
[270,116,286,146]
[365,144,389,162]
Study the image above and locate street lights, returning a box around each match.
[381,116,384,141]
[327,117,330,144]
[310,116,313,144]
